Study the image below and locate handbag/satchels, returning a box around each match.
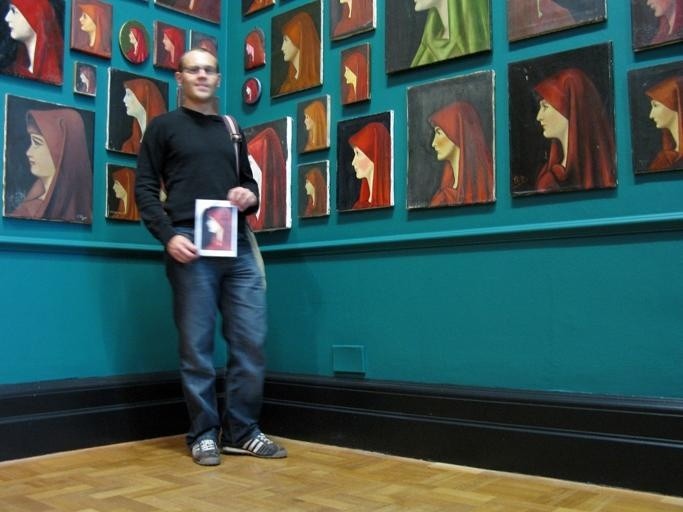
[224,116,268,290]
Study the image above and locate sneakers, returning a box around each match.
[220,432,287,461]
[185,434,220,468]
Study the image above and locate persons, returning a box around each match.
[242,80,257,104]
[109,166,138,222]
[160,27,185,69]
[135,50,289,465]
[9,108,90,224]
[531,71,613,192]
[77,0,112,57]
[646,71,682,172]
[410,1,492,64]
[303,168,328,218]
[127,23,149,63]
[347,122,391,207]
[302,102,327,148]
[277,10,319,90]
[200,206,232,251]
[344,53,369,106]
[332,0,372,35]
[646,0,681,43]
[246,33,264,70]
[248,127,283,230]
[79,64,95,95]
[428,100,493,206]
[121,74,169,156]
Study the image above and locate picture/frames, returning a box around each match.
[298,160,330,219]
[341,42,372,106]
[119,22,151,65]
[631,0,682,53]
[241,0,275,17]
[4,94,95,225]
[270,0,324,98]
[106,162,142,221]
[245,30,267,70]
[106,67,169,156]
[241,116,293,233]
[508,0,607,44]
[385,0,492,76]
[72,1,114,60]
[74,62,97,96]
[297,94,331,155]
[153,20,187,71]
[155,0,220,25]
[627,61,682,175]
[242,78,263,104]
[406,70,495,211]
[337,110,394,212]
[195,199,238,256]
[508,40,618,197]
[190,31,217,58]
[330,10,377,40]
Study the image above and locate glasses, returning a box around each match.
[179,68,216,77]
